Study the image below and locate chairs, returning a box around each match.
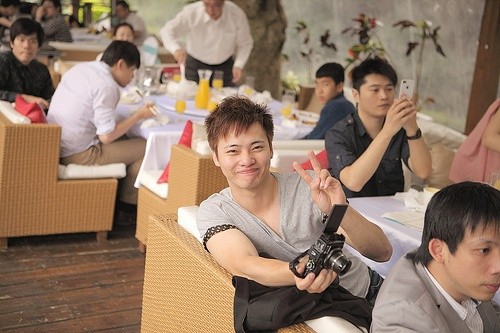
[1,100,128,253]
[299,85,323,115]
[270,137,330,169]
[135,146,230,253]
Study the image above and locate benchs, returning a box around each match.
[140,205,368,332]
[46,28,174,86]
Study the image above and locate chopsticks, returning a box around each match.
[135,90,160,116]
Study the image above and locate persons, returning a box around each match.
[0,0,20,38]
[370,180,500,333]
[326,57,432,198]
[161,0,254,88]
[299,62,355,140]
[113,1,147,42]
[48,40,158,226]
[196,95,393,307]
[35,0,72,46]
[96,25,146,87]
[0,17,55,115]
[449,99,500,187]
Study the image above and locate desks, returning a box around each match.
[113,84,321,188]
[338,193,500,307]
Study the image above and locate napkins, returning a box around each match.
[393,188,425,207]
[382,211,424,231]
[141,114,169,129]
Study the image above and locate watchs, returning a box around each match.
[406,127,422,141]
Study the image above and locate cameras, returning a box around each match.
[298,204,355,278]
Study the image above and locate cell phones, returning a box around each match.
[399,79,413,103]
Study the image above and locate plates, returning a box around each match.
[294,109,321,123]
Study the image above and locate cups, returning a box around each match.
[245,76,255,95]
[212,70,224,93]
[173,69,181,82]
[284,90,296,106]
[282,95,293,119]
[423,187,440,203]
[175,89,186,113]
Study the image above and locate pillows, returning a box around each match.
[14,95,48,124]
[157,120,193,183]
[294,150,330,169]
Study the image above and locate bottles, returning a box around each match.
[195,70,213,109]
[135,65,168,95]
